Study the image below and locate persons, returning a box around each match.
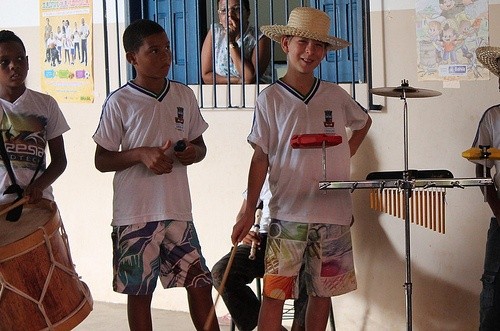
[43,17,90,66]
[0,30,71,205]
[231,6,372,331]
[200,0,271,85]
[211,174,354,331]
[470,45,500,331]
[91,18,220,331]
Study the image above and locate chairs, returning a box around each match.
[230,275,337,331]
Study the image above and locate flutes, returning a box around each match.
[249,200,264,260]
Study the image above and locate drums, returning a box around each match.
[0,196,93,331]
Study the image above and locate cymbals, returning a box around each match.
[368,87,442,98]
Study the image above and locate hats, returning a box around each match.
[260,7,352,51]
[475,46,500,77]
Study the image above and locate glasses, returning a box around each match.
[217,5,246,14]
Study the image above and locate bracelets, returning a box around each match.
[229,42,238,48]
